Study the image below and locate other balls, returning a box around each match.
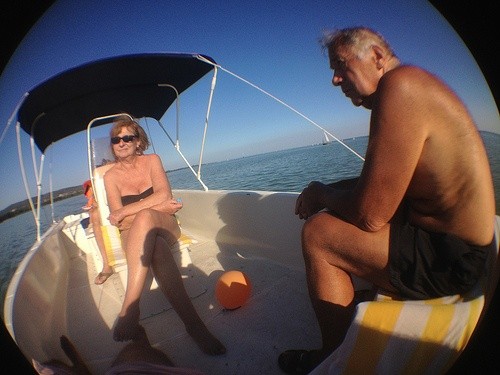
[216,270,253,310]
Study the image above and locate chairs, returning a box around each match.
[313,213,500,375]
[87,113,207,320]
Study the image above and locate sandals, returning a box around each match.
[278,349,322,374]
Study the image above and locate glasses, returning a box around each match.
[110,135,138,144]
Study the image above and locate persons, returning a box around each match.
[277,28,496,375]
[32,119,228,375]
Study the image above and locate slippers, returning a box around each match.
[95,266,115,285]
